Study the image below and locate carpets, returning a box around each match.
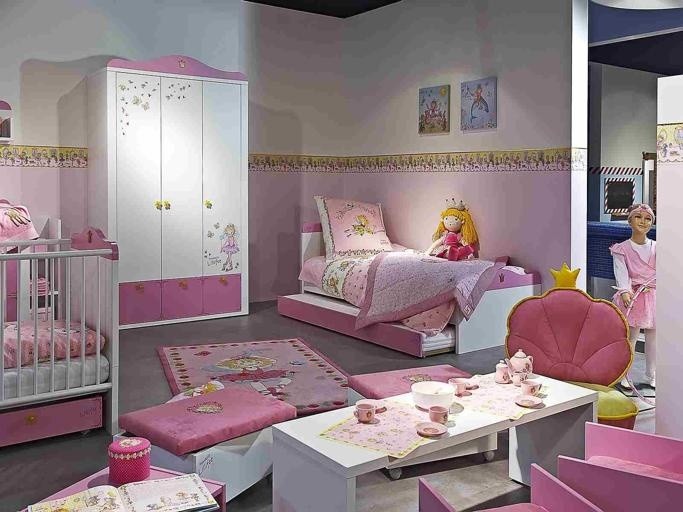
[153,334,352,418]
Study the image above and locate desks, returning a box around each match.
[16,464,226,511]
[269,370,599,512]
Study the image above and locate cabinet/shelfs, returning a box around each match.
[83,54,249,331]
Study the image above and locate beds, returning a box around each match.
[275,221,544,362]
[0,215,120,449]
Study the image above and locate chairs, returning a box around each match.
[502,261,641,431]
[556,419,682,512]
[416,461,603,512]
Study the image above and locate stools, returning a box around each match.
[343,361,499,477]
[106,377,297,505]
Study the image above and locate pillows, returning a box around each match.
[311,194,394,263]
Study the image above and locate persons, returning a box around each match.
[425,196,478,261]
[608,201,655,390]
